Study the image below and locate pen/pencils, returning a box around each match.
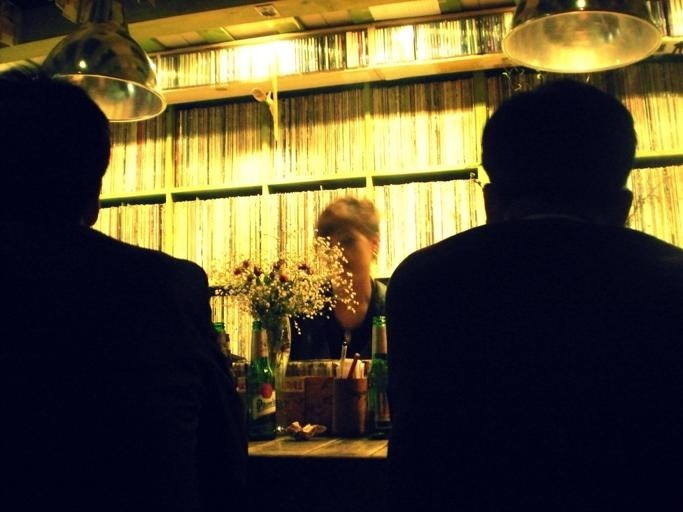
[340,342,359,379]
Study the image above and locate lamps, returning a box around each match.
[41,0,167,124]
[500,0,667,74]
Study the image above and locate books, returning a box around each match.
[98,72,515,195]
[516,60,682,152]
[150,10,516,89]
[90,176,486,366]
[627,165,682,248]
[656,1,682,55]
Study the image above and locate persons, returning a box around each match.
[0,60,249,510]
[383,77,683,509]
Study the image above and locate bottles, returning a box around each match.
[365,317,389,440]
[245,320,275,440]
[214,321,231,361]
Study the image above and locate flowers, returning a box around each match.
[203,220,361,351]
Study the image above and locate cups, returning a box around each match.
[304,376,367,436]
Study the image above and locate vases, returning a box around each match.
[254,316,294,379]
